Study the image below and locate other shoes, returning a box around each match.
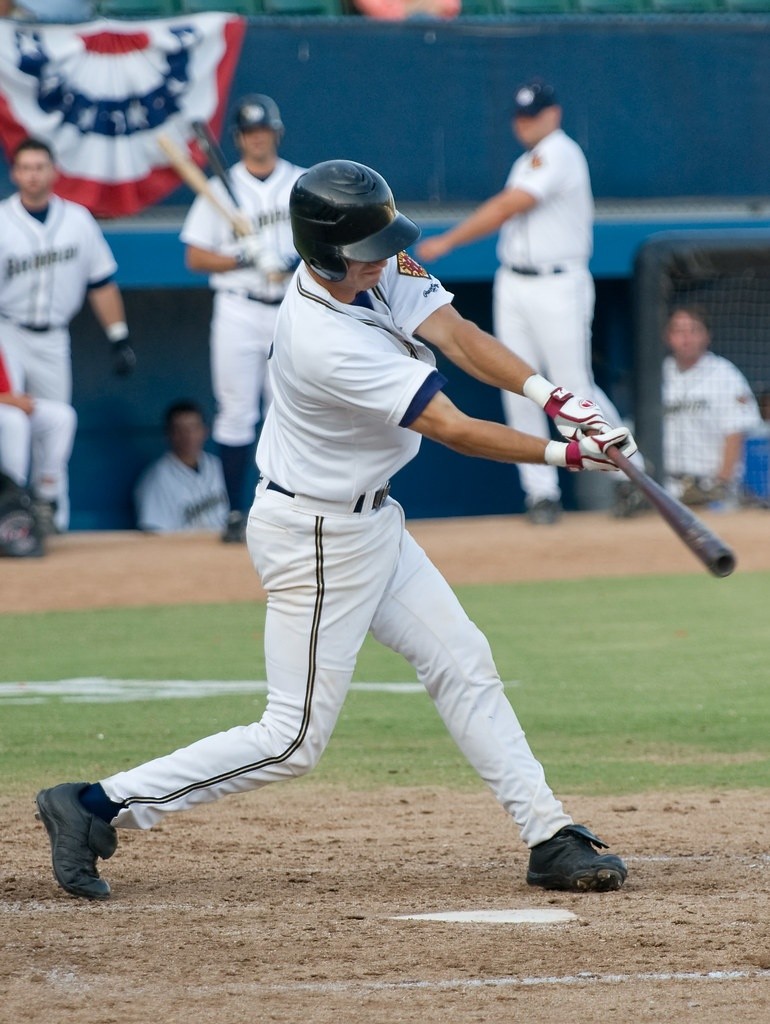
[614,457,655,517]
[530,500,560,524]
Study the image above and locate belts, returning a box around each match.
[16,319,55,333]
[247,291,285,307]
[266,481,388,512]
[515,265,562,276]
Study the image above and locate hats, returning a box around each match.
[238,96,283,131]
[511,81,558,118]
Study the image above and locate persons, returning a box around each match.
[178,95,308,541]
[35,160,637,900]
[661,306,766,510]
[0,136,135,531]
[136,400,229,531]
[417,79,651,525]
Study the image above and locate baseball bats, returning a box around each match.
[588,428,736,577]
[155,129,285,284]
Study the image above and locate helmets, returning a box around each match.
[291,161,420,282]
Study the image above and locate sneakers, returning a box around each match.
[36,781,119,899]
[528,822,628,893]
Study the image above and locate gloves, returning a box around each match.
[544,427,637,474]
[521,376,611,442]
[111,338,138,376]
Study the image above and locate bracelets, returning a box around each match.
[106,322,129,341]
